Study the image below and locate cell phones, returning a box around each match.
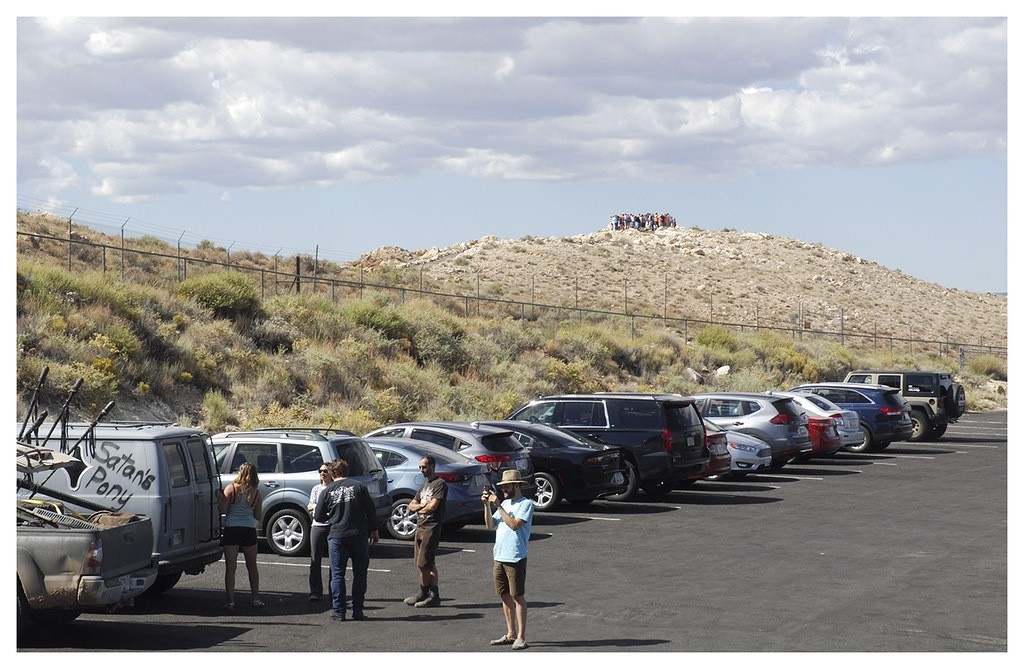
[483,484,491,501]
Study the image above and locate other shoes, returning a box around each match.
[490,635,514,644]
[309,595,321,601]
[330,610,344,621]
[413,596,441,608]
[353,615,368,620]
[511,639,526,650]
[404,590,428,605]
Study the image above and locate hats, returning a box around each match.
[497,470,528,484]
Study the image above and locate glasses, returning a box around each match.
[318,469,328,473]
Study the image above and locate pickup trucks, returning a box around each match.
[17,442,162,630]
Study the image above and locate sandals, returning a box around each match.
[254,600,267,608]
[226,601,235,609]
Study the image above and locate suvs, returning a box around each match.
[504,390,711,499]
[17,420,225,593]
[843,369,965,442]
[205,428,393,556]
[788,382,914,452]
[364,421,538,525]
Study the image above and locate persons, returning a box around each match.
[219,462,265,609]
[404,455,448,608]
[314,459,379,622]
[481,470,533,649]
[307,462,334,603]
[610,212,676,231]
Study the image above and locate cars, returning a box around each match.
[470,419,629,512]
[690,392,814,482]
[763,391,864,459]
[359,436,497,541]
